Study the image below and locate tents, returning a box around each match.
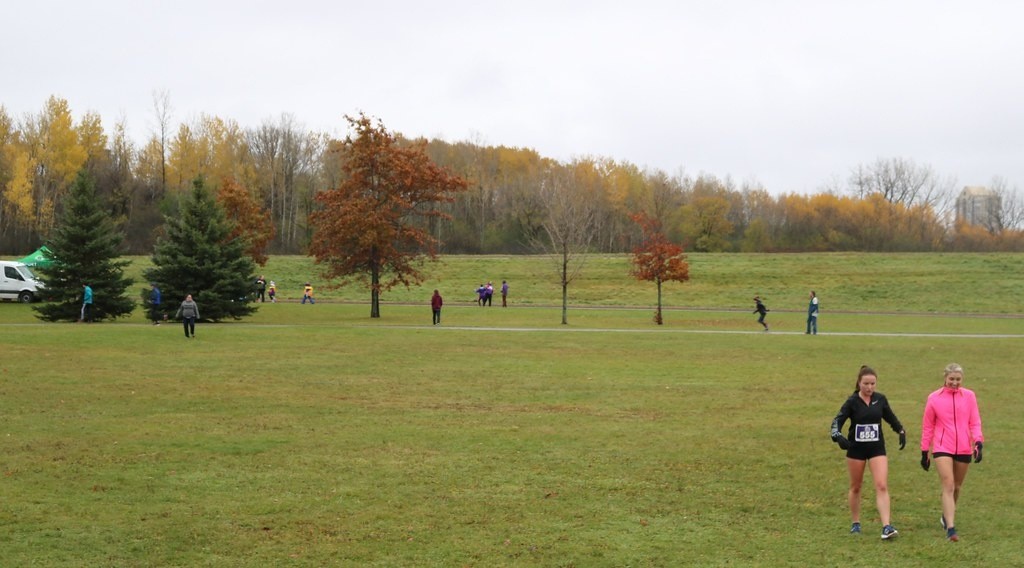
[20,244,69,281]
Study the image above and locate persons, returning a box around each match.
[254,275,267,304]
[921,363,983,541]
[76,281,94,324]
[752,296,770,332]
[806,291,819,334]
[146,283,168,326]
[267,281,277,303]
[175,294,200,339]
[830,366,906,541]
[474,284,486,306]
[431,290,442,326]
[483,281,493,307]
[301,283,315,305]
[501,280,508,307]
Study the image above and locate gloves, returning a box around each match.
[921,450,930,471]
[899,430,907,450]
[836,435,852,449]
[973,441,983,463]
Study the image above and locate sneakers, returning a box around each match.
[881,525,898,539]
[849,523,861,533]
[946,528,958,542]
[940,515,947,529]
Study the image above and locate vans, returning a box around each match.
[0,260,51,304]
